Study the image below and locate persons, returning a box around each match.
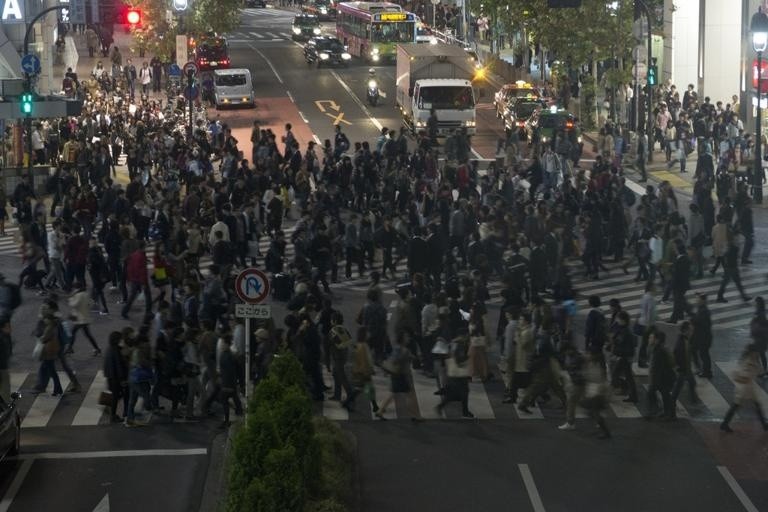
[1,3,766,434]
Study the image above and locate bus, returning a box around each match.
[335,1,417,64]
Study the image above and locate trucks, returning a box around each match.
[393,43,477,151]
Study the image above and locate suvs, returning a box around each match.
[292,14,322,40]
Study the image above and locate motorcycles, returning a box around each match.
[366,80,380,106]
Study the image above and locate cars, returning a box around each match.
[415,14,482,70]
[300,0,337,21]
[493,79,585,159]
[303,36,353,68]
[0,390,23,464]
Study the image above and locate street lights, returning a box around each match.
[749,4,768,205]
[173,0,188,35]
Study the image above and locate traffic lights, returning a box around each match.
[648,66,657,86]
[22,93,30,113]
[103,10,141,25]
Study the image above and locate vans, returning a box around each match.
[193,24,258,109]
